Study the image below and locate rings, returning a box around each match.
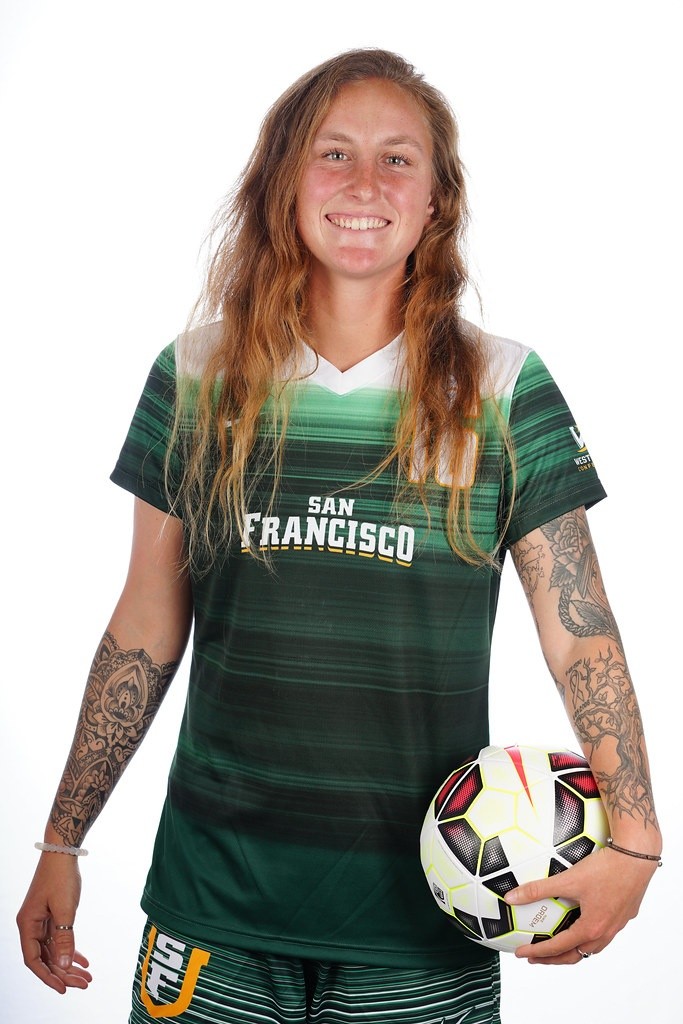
[56,925,73,930]
[46,937,53,945]
[577,949,590,958]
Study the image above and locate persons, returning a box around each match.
[16,50,662,1024]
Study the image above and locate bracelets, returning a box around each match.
[34,842,88,856]
[606,838,662,866]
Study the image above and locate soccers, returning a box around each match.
[419,744,611,953]
